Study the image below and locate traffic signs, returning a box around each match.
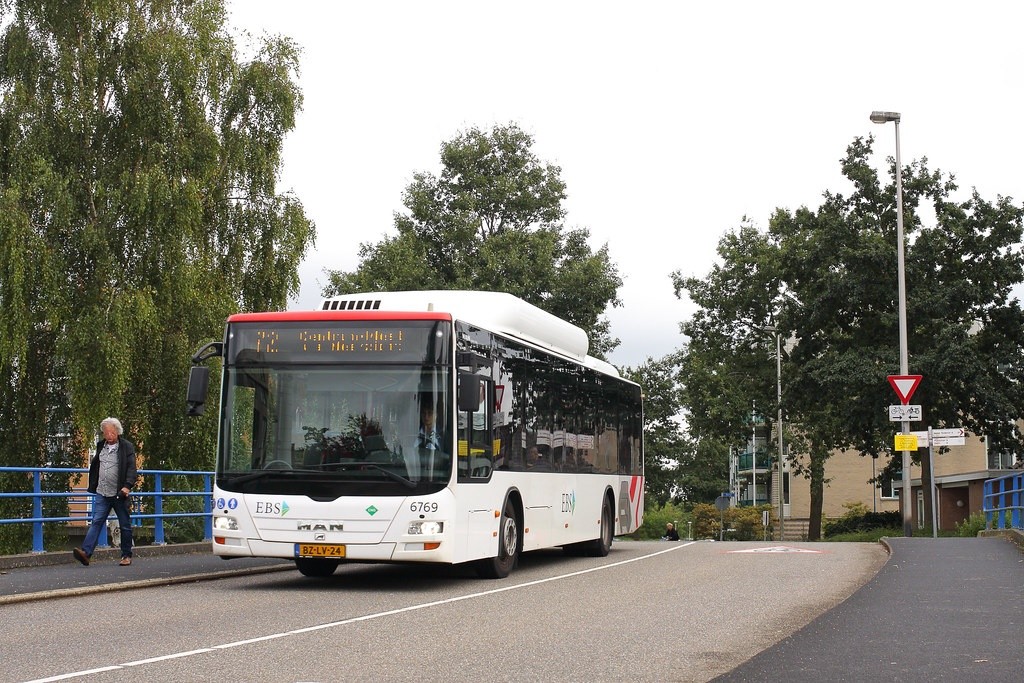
[932,428,964,437]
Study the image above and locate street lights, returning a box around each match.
[674,521,677,530]
[869,110,913,537]
[688,522,692,541]
[763,326,784,542]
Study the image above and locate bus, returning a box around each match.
[184,290,645,578]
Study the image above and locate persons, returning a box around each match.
[413,403,444,453]
[524,445,564,472]
[661,523,679,541]
[73,417,137,567]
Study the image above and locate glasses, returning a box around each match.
[421,411,436,417]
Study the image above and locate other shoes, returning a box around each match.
[119,557,132,565]
[73,549,90,566]
